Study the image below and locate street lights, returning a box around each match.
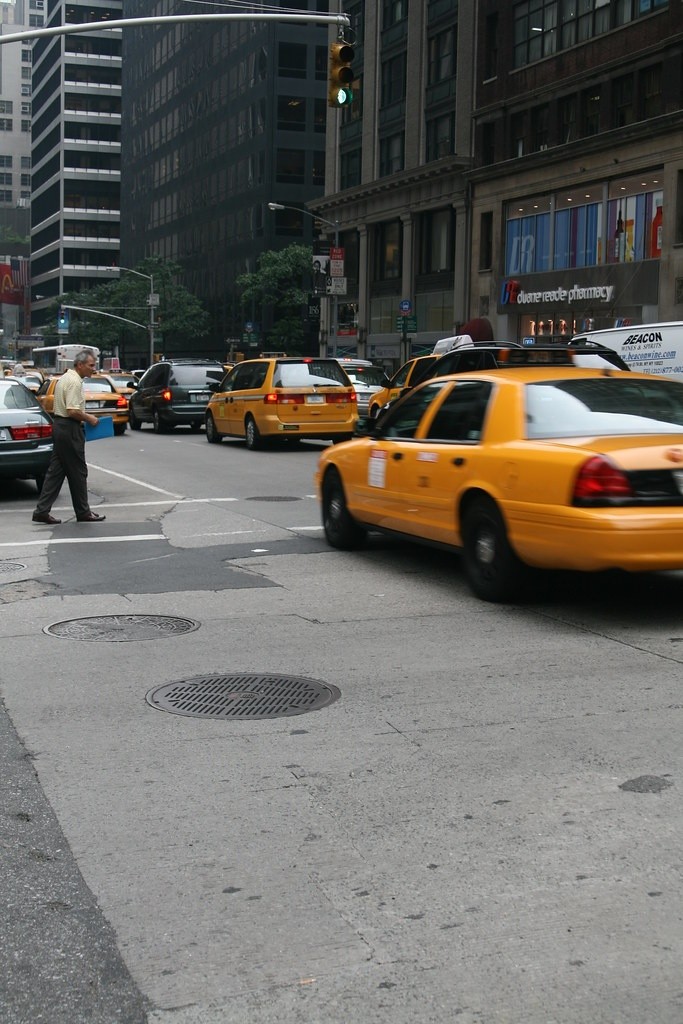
[268,202,350,365]
[108,266,161,370]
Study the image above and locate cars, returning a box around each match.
[0,359,48,394]
[95,371,141,404]
[333,356,396,417]
[36,375,127,437]
[317,370,683,604]
[0,378,55,491]
[205,357,361,450]
[364,333,476,423]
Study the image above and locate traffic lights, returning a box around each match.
[60,311,67,326]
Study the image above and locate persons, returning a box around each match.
[32,351,105,524]
[313,260,327,291]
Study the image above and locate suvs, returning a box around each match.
[375,337,631,426]
[128,357,228,434]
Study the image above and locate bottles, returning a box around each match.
[651,197,662,258]
[613,211,625,263]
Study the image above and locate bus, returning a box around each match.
[31,344,101,380]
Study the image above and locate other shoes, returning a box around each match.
[32,514,61,524]
[77,511,105,521]
